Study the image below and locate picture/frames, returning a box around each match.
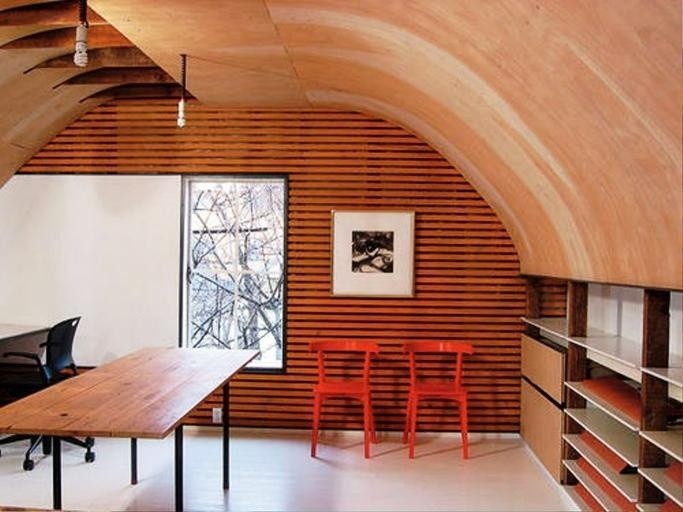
[331,208,416,299]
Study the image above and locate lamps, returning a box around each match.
[177,54,187,127]
[72,0,89,67]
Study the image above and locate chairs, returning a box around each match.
[1,316,96,471]
[402,339,475,460]
[307,339,380,458]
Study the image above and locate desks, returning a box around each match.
[0,347,260,512]
[0,323,51,364]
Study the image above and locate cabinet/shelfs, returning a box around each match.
[521,314,566,482]
[560,332,683,511]
